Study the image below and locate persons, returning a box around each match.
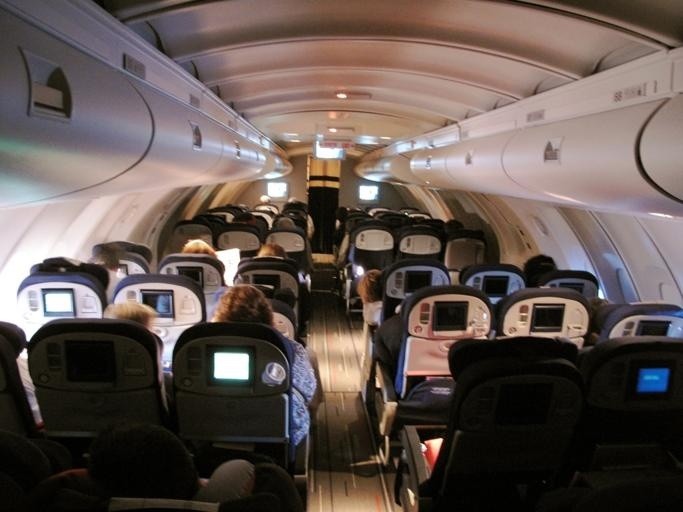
[234,195,305,233]
[334,207,348,243]
[115,300,172,402]
[275,285,296,308]
[257,245,287,258]
[211,285,316,450]
[359,271,383,367]
[91,245,127,301]
[183,240,218,259]
[75,427,304,512]
[525,253,555,285]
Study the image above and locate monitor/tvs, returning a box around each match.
[634,321,671,336]
[624,359,676,400]
[433,302,468,331]
[559,283,584,295]
[530,304,566,332]
[356,181,379,205]
[63,339,116,386]
[267,180,288,202]
[118,265,128,275]
[495,382,554,425]
[482,275,509,297]
[205,343,257,388]
[176,266,204,290]
[139,288,175,320]
[251,274,281,291]
[41,289,77,318]
[313,140,345,161]
[404,271,432,293]
